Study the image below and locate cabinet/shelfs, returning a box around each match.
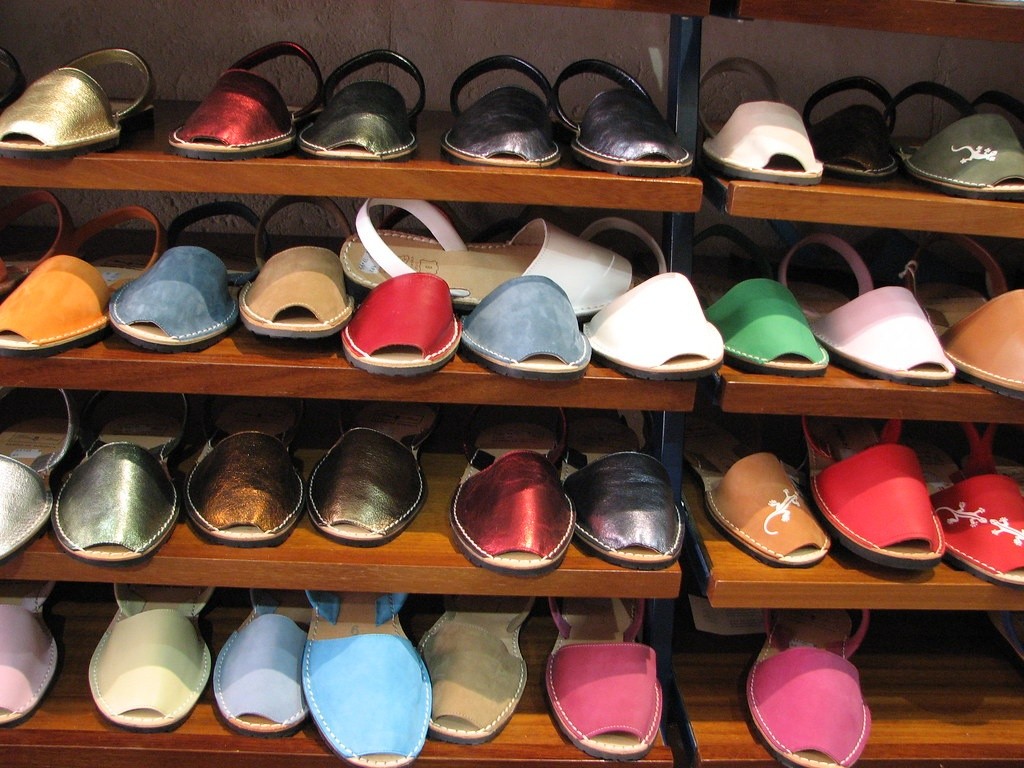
[652,0,1024,768]
[0,0,712,768]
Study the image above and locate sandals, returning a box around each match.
[1,38,1024,768]
